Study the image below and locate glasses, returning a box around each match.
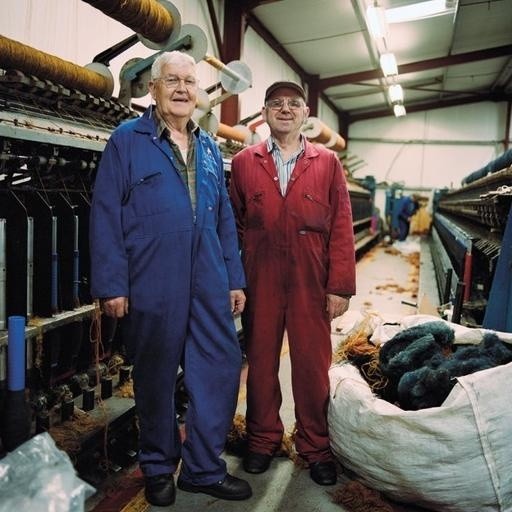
[153,75,196,89]
[267,101,301,110]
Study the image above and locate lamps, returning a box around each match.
[366,0,457,119]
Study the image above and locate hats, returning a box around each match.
[264,80,307,106]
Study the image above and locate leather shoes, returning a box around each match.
[242,452,274,473]
[142,474,252,507]
[310,461,337,485]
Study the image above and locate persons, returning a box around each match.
[225,77,360,489]
[395,191,422,240]
[86,49,254,509]
